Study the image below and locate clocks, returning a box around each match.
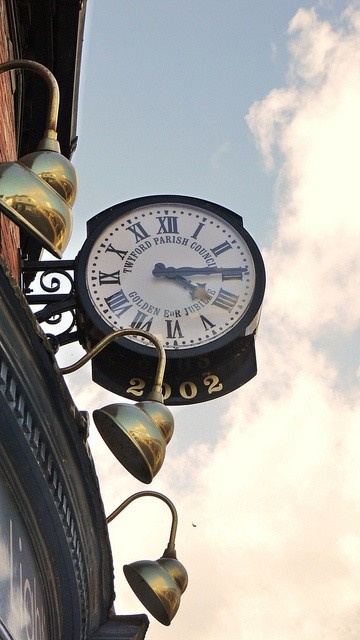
[71,191,265,404]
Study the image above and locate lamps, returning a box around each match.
[59,328,174,486]
[105,489,188,627]
[0,56,78,258]
